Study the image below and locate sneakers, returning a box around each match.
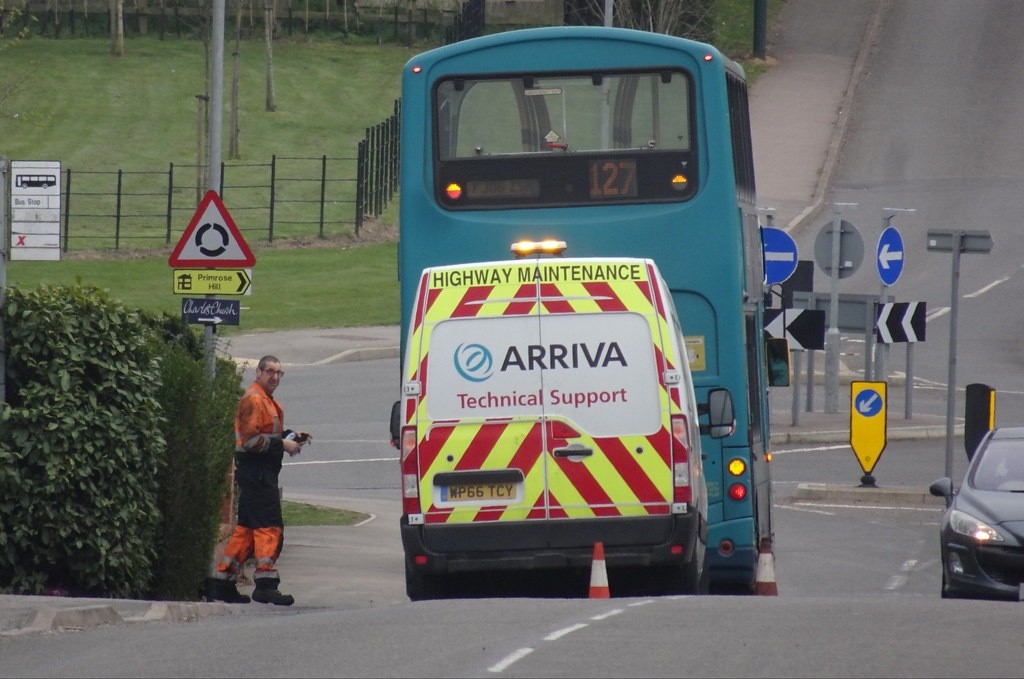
[252,578,294,605]
[206,579,251,604]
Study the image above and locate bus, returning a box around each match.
[398,27,790,595]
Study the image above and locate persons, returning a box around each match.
[217,355,313,607]
[769,347,789,386]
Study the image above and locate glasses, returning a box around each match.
[261,369,285,378]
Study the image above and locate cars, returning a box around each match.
[929,427,1024,600]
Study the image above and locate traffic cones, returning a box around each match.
[752,537,778,596]
[586,543,611,598]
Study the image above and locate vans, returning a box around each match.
[390,240,736,599]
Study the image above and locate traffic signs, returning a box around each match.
[173,268,251,296]
[181,297,240,325]
